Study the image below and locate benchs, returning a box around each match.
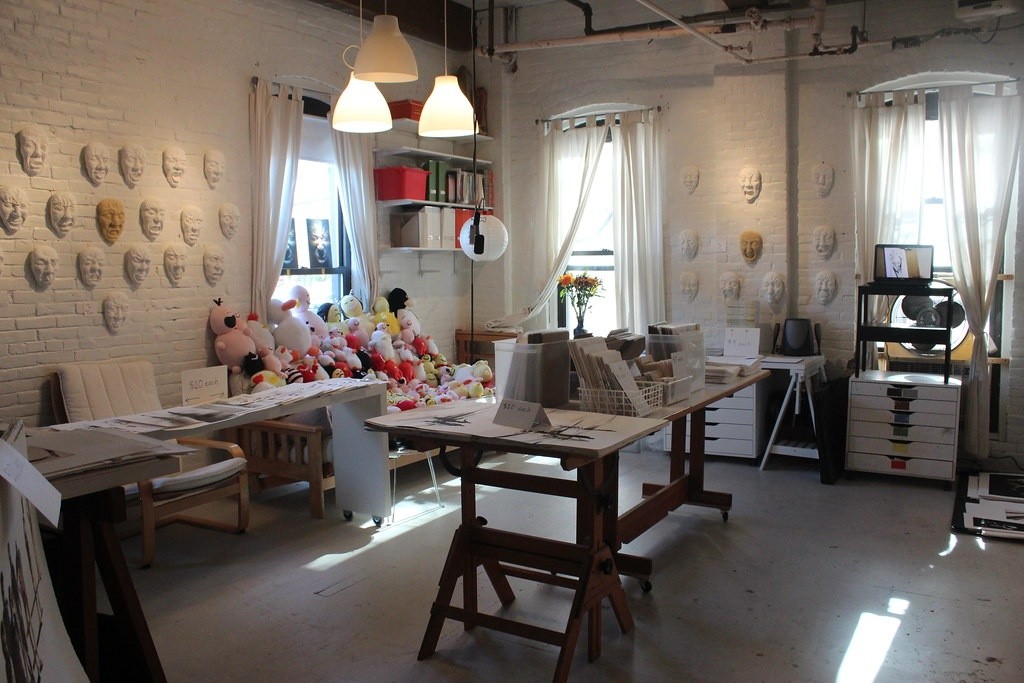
[226,421,509,521]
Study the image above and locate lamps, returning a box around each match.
[331,0,392,133]
[342,0,419,83]
[459,199,509,260]
[418,0,480,138]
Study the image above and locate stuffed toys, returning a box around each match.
[210,298,257,375]
[224,286,496,414]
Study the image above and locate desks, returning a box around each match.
[35,427,181,683]
[363,370,771,683]
[760,355,826,471]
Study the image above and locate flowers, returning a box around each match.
[556,271,606,318]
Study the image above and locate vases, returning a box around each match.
[574,317,587,336]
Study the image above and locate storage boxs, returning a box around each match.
[491,338,570,406]
[388,99,422,121]
[646,329,707,393]
[373,165,432,200]
[664,375,693,406]
[576,381,662,418]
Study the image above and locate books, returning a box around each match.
[421,159,494,208]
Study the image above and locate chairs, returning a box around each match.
[49,360,250,568]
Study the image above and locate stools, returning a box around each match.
[455,329,519,365]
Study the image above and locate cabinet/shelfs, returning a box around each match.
[855,281,955,385]
[845,368,962,490]
[373,118,494,277]
[663,377,769,458]
